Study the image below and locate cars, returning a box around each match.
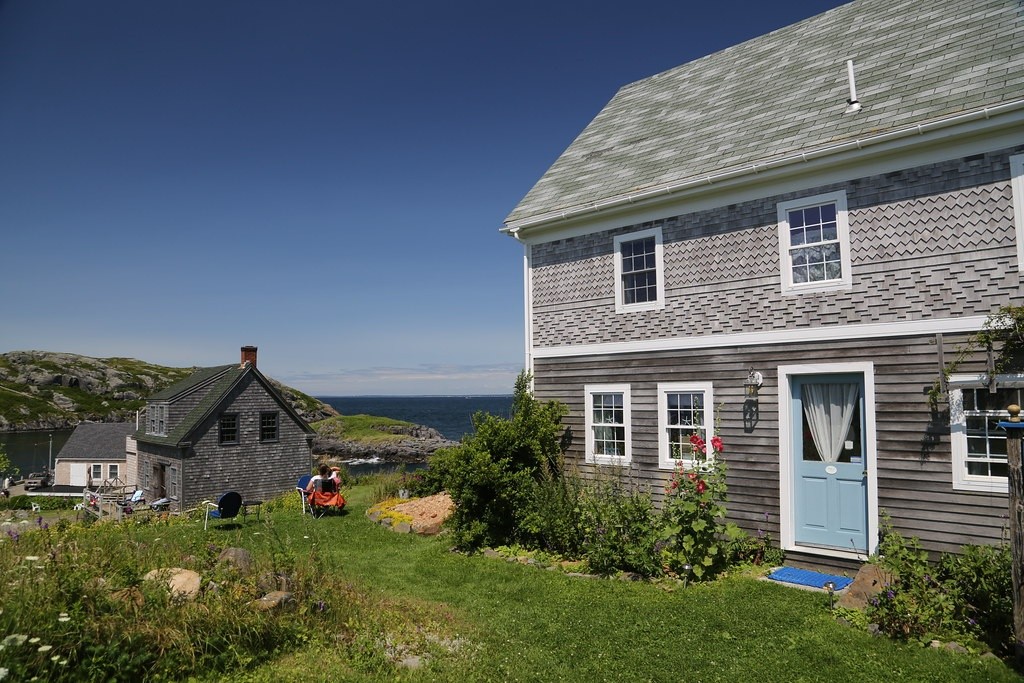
[24,472,49,490]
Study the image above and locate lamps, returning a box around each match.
[741,365,760,404]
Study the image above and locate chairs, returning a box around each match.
[296,475,314,510]
[297,479,335,519]
[202,490,242,531]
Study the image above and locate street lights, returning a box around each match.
[48,433,53,475]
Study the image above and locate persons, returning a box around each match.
[306,463,341,492]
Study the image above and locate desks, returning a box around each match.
[241,499,264,526]
